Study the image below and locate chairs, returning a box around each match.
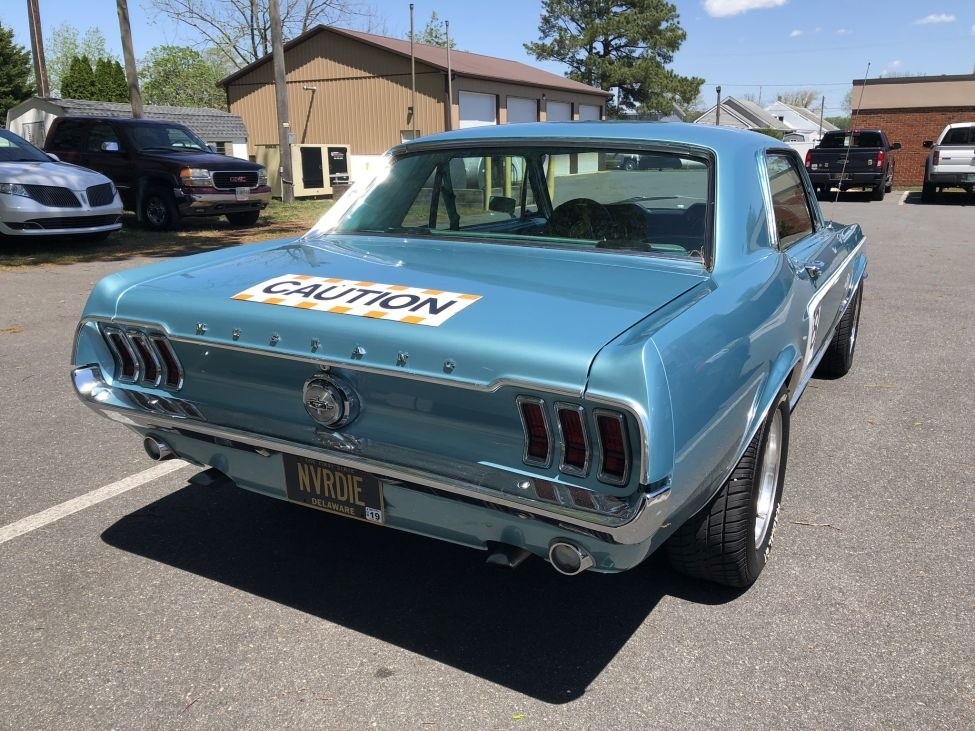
[543,195,802,251]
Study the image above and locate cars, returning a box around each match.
[69,119,869,589]
[0,128,123,245]
[604,153,644,171]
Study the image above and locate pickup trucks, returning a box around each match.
[805,127,902,201]
[922,122,975,201]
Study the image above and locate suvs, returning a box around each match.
[43,115,272,231]
